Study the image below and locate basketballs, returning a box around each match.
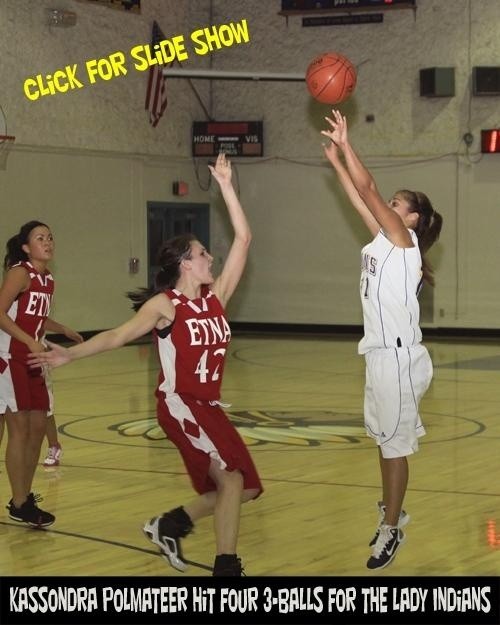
[306,52,356,104]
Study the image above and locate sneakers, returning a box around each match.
[366,501,410,571]
[213,556,243,581]
[142,506,195,573]
[6,492,55,528]
[44,443,62,466]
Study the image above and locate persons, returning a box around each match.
[0,220,83,528]
[321,109,443,570]
[26,152,264,577]
